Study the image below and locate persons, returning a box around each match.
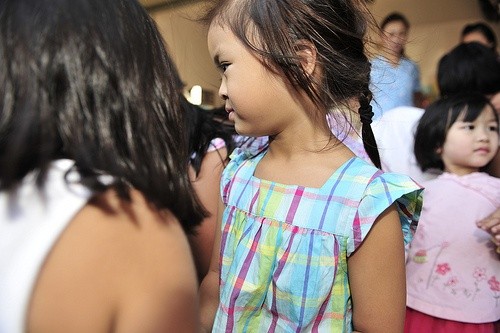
[0,1,500,333]
